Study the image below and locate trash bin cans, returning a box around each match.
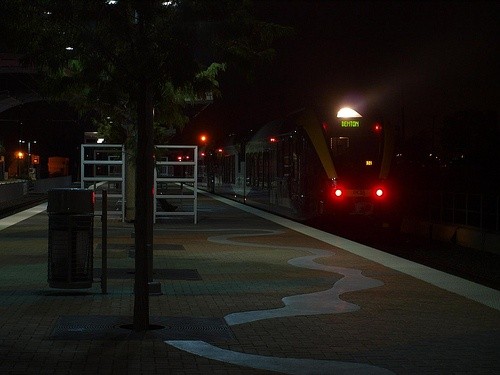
[44,187,95,290]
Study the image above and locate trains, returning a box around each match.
[166,99,407,235]
